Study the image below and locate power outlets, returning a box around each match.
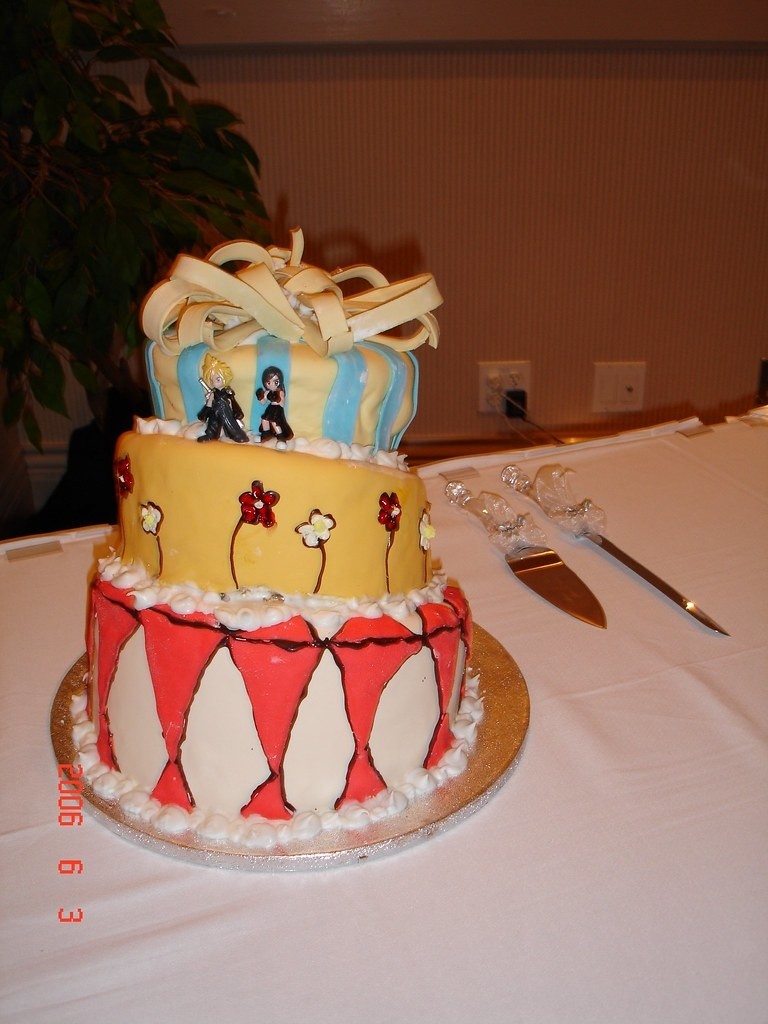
[477,360,531,413]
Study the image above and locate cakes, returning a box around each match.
[68,225,485,848]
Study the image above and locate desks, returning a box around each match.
[0,398,768,1024]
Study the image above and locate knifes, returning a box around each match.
[499,466,735,637]
